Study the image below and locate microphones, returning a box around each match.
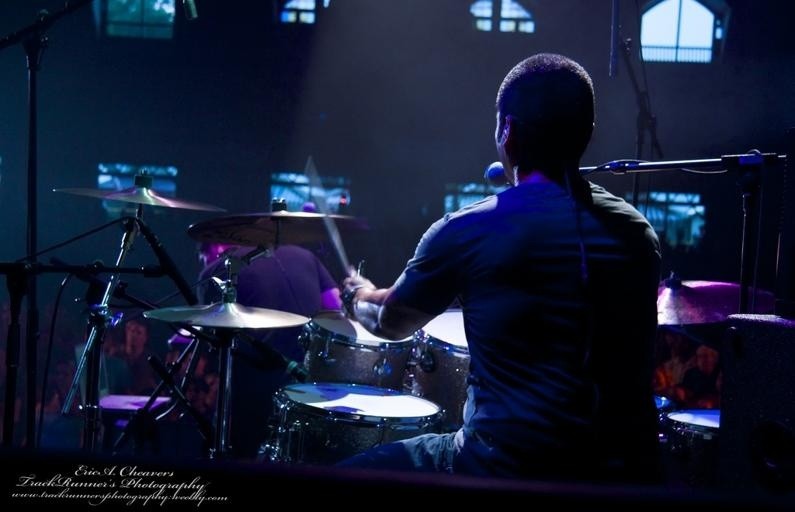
[484,160,509,187]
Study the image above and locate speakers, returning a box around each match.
[720,312,795,499]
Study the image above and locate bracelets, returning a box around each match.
[344,282,369,318]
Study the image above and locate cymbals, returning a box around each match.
[144,302,313,329]
[52,184,227,213]
[186,210,360,243]
[656,280,776,326]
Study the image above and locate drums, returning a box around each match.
[257,380,447,468]
[658,407,722,473]
[653,394,678,435]
[402,306,472,430]
[297,307,433,398]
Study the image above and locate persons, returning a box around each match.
[0,295,218,469]
[670,342,721,411]
[327,50,668,487]
[194,240,346,460]
